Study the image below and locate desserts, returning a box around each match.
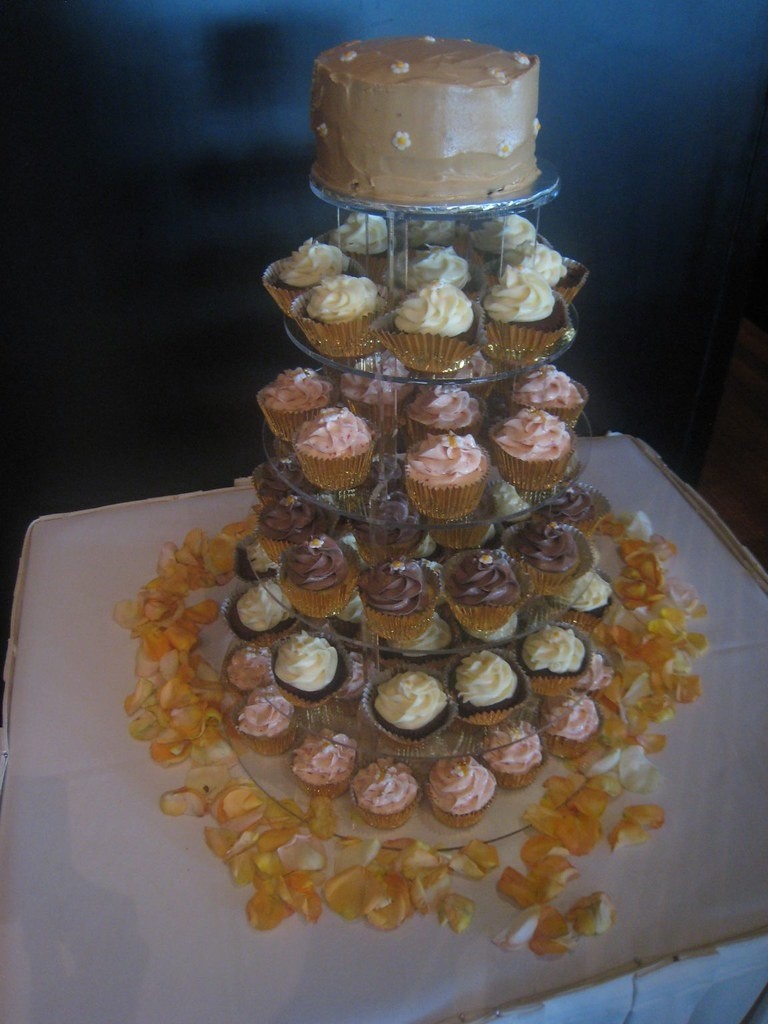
[226,212,611,830]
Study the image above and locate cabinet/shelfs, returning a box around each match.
[205,155,620,852]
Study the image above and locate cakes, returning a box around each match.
[310,35,543,206]
